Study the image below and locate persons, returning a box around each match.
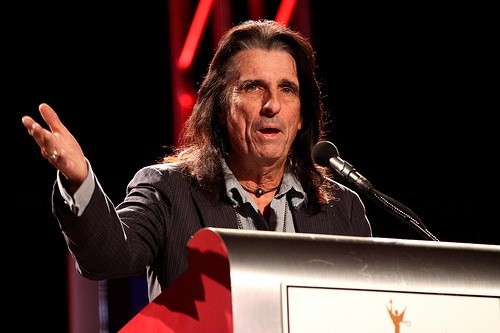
[22,19,373,304]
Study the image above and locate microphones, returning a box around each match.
[311,141,373,192]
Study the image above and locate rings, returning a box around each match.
[45,149,57,160]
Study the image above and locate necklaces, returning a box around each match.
[241,184,281,198]
[235,195,288,232]
